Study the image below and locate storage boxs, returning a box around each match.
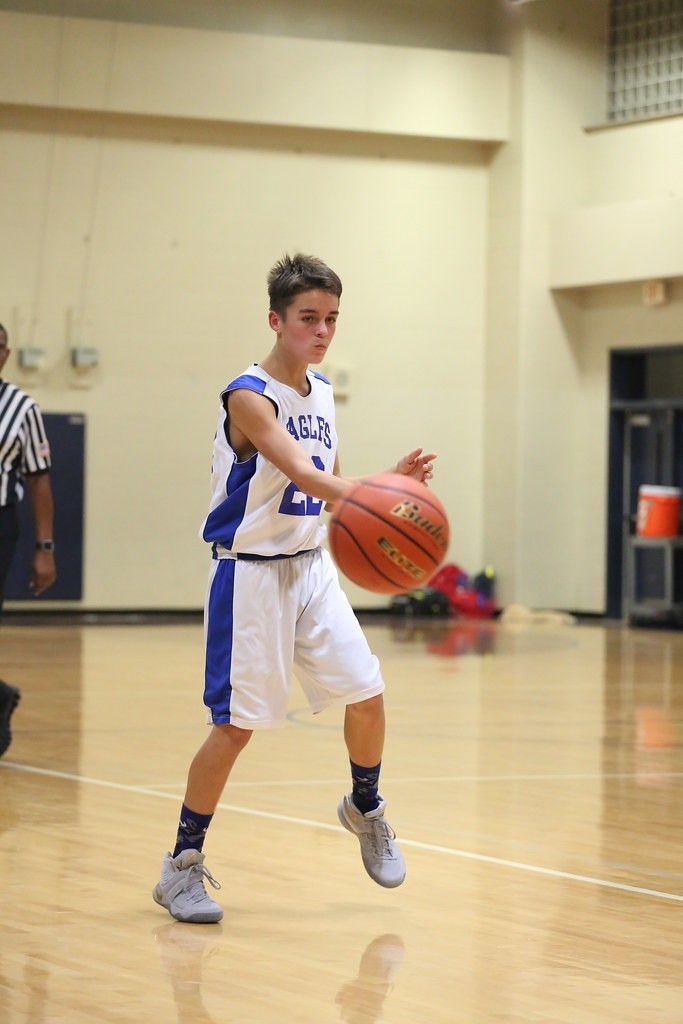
[636,484,682,539]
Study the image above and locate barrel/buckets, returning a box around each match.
[636,483,681,538]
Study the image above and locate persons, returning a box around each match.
[0,322,57,758]
[153,252,437,924]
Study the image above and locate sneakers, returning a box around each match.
[152,849,224,923]
[338,793,406,888]
[0,681,21,760]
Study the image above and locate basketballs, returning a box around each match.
[328,473,451,596]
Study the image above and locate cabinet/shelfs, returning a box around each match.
[629,538,682,630]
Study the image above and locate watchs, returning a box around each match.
[36,541,54,552]
